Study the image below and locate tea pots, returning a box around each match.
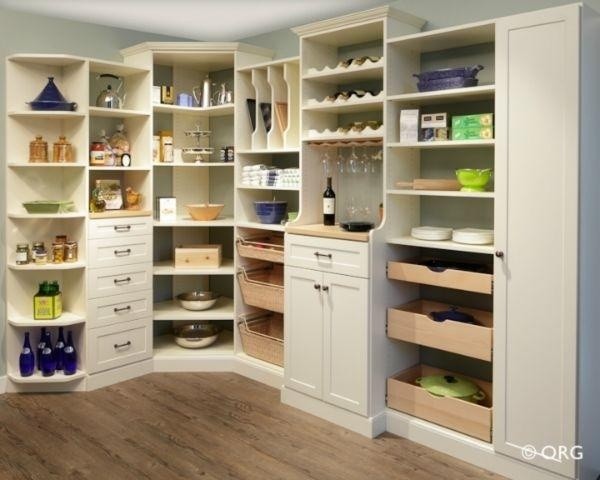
[95,73,127,110]
[193,73,217,108]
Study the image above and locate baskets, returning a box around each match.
[236,235,284,264]
[236,267,284,314]
[237,315,284,367]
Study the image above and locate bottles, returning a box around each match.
[16,234,77,264]
[163,141,173,162]
[19,328,78,376]
[91,129,132,166]
[153,135,161,162]
[323,176,336,226]
[28,135,50,163]
[177,93,192,106]
[33,281,62,320]
[217,81,233,104]
[51,136,73,162]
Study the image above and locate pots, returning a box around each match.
[416,371,485,404]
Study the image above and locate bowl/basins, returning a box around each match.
[186,204,223,221]
[412,62,486,92]
[175,289,218,311]
[169,324,224,348]
[253,200,288,223]
[455,168,492,186]
[23,199,75,213]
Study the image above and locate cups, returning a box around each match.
[226,147,233,161]
[220,149,228,162]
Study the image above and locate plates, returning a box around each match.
[340,221,375,231]
[411,225,494,246]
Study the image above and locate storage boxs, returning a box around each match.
[175,244,223,268]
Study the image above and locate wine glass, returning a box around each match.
[126,188,140,210]
[319,141,380,176]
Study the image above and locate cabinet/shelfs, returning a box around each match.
[5,53,153,383]
[119,41,275,356]
[387,3,600,480]
[284,4,429,419]
[234,56,301,372]
